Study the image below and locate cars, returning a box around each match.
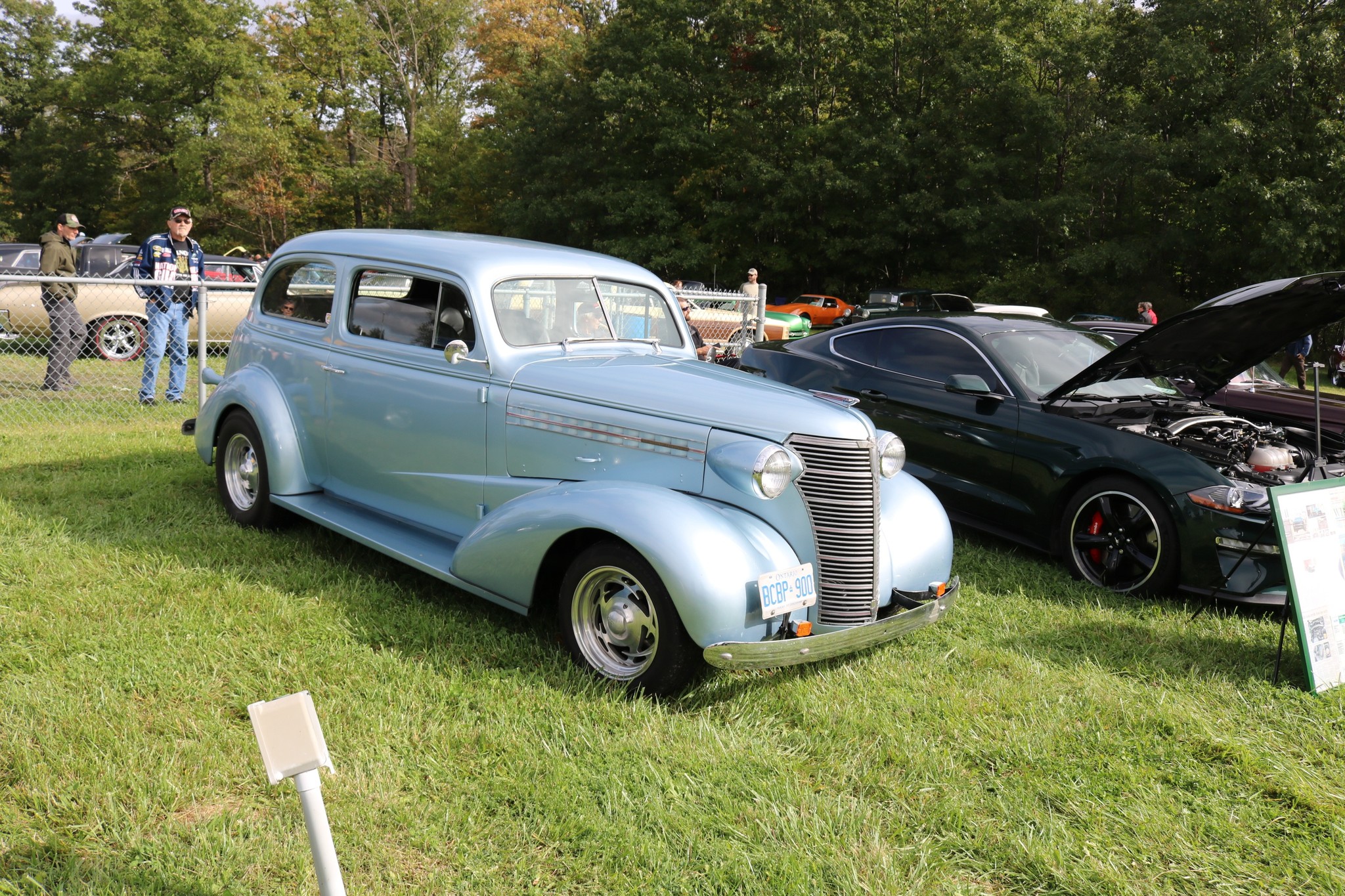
[735,271,1345,605]
[508,281,855,360]
[181,229,965,705]
[0,233,334,362]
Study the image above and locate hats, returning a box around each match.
[746,268,758,275]
[170,206,191,220]
[77,232,86,237]
[57,213,86,229]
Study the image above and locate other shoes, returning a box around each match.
[140,398,157,407]
[44,383,62,391]
[173,398,187,405]
[60,374,80,386]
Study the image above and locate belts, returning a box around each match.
[172,297,188,304]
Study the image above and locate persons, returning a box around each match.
[249,253,272,266]
[282,298,298,317]
[1137,302,1157,324]
[131,206,208,407]
[576,302,602,338]
[1278,334,1313,391]
[39,213,86,391]
[671,280,720,362]
[734,268,759,315]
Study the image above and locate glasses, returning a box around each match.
[285,306,294,311]
[682,306,689,311]
[171,219,191,224]
[1137,307,1141,309]
[747,274,754,277]
